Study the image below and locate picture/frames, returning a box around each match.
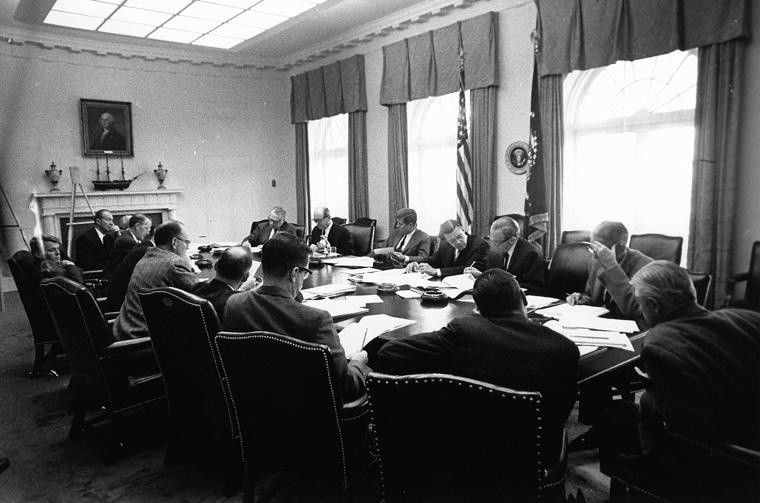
[80,98,135,158]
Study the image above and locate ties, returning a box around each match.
[397,235,406,250]
[322,229,325,238]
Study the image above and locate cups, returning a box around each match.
[329,246,337,255]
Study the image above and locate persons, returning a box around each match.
[377,268,581,485]
[30,235,83,375]
[188,244,252,329]
[113,220,256,342]
[565,220,655,320]
[462,216,546,297]
[403,220,490,278]
[309,207,355,256]
[76,209,156,313]
[382,208,431,268]
[628,259,760,503]
[89,112,126,151]
[221,231,371,404]
[241,207,296,247]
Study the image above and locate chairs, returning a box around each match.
[6,217,760,503]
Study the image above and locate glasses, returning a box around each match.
[176,237,190,246]
[487,234,510,246]
[299,266,312,280]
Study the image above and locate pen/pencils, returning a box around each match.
[360,329,370,353]
[565,292,585,305]
[464,260,477,275]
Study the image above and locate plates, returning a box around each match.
[191,244,223,268]
